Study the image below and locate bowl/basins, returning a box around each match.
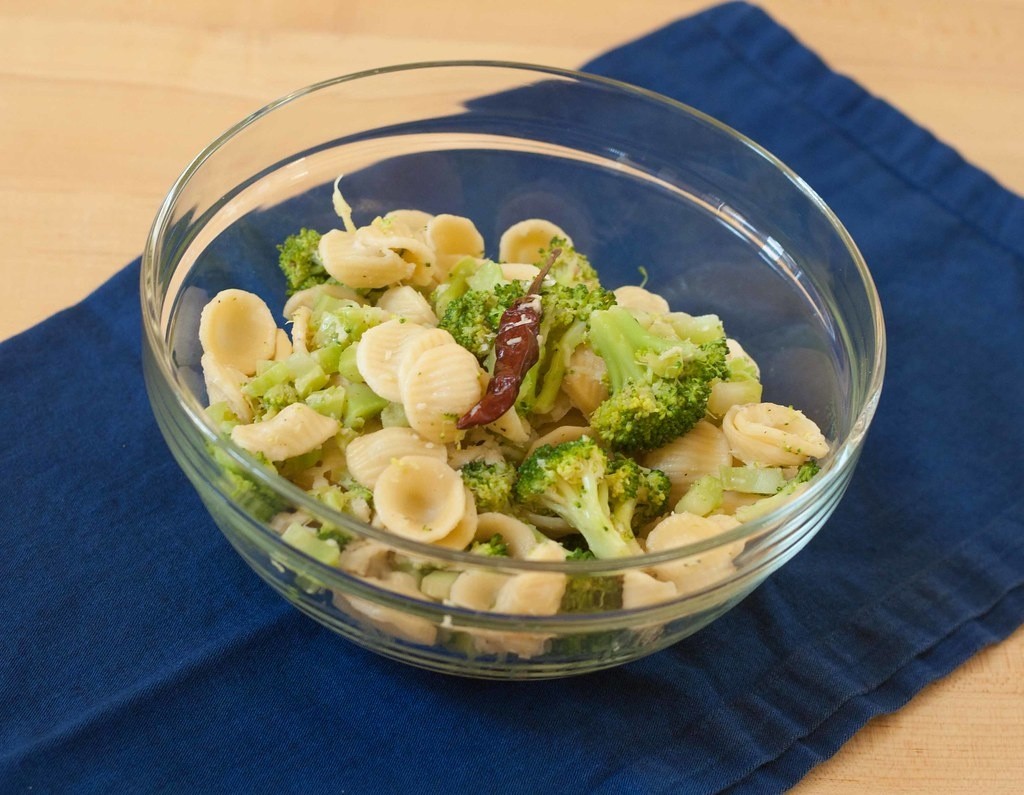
[141,62,886,679]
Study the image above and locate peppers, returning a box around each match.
[457,249,565,429]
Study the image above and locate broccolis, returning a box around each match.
[202,228,817,656]
